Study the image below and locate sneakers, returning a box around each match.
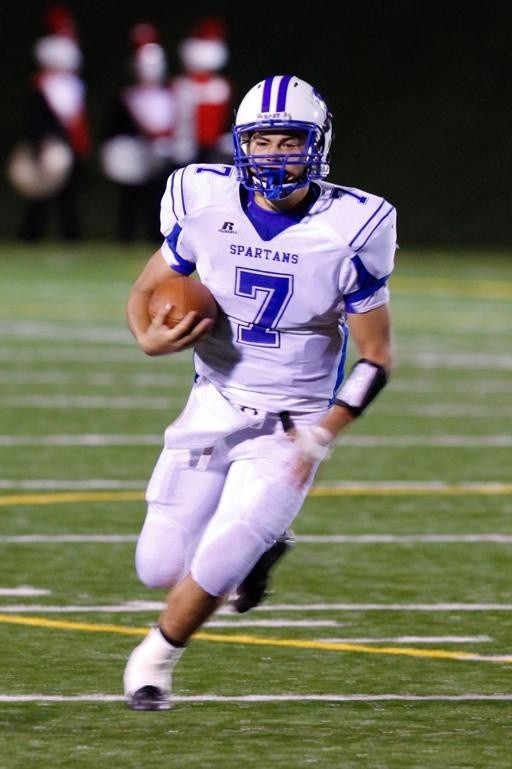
[232,532,291,613]
[124,651,175,709]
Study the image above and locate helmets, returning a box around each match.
[229,73,336,202]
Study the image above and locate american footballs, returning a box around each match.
[147,276,222,342]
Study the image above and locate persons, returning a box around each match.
[8,10,233,245]
[121,71,401,710]
